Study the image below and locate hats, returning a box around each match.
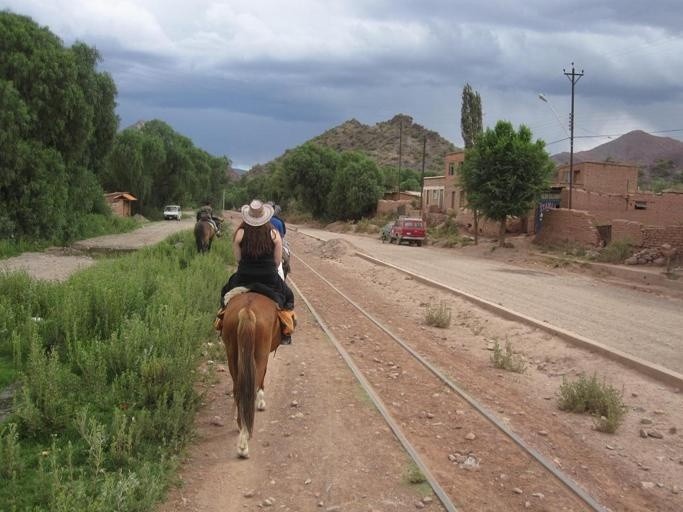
[241,200,274,227]
[267,200,280,216]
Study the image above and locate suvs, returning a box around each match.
[388,217,426,247]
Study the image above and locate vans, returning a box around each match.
[163,205,182,221]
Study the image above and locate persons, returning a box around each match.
[196,199,297,345]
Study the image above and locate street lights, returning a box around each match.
[537,93,573,210]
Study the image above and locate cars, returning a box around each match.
[382,222,396,242]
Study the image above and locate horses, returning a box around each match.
[279,241,290,278]
[216,290,294,460]
[193,219,219,256]
